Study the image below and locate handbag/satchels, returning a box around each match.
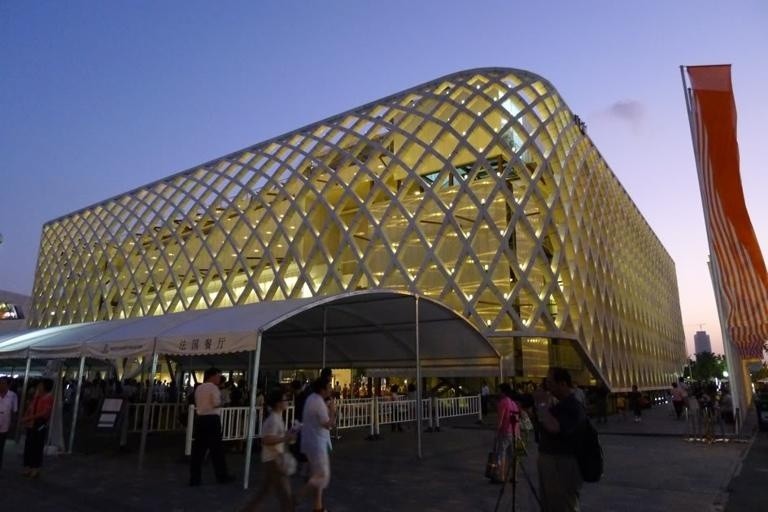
[34,416,48,433]
[277,451,298,476]
[485,450,506,481]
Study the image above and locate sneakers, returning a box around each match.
[21,469,42,480]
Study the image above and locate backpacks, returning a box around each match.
[574,413,606,482]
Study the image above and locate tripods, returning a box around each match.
[493,414,541,512]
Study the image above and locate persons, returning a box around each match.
[670,375,735,436]
[626,384,643,424]
[187,367,236,486]
[499,376,557,447]
[15,376,56,479]
[570,379,588,409]
[290,375,336,512]
[187,379,491,416]
[491,387,520,484]
[237,391,301,512]
[592,378,613,426]
[62,376,167,433]
[533,364,609,512]
[0,378,18,470]
[501,400,529,479]
